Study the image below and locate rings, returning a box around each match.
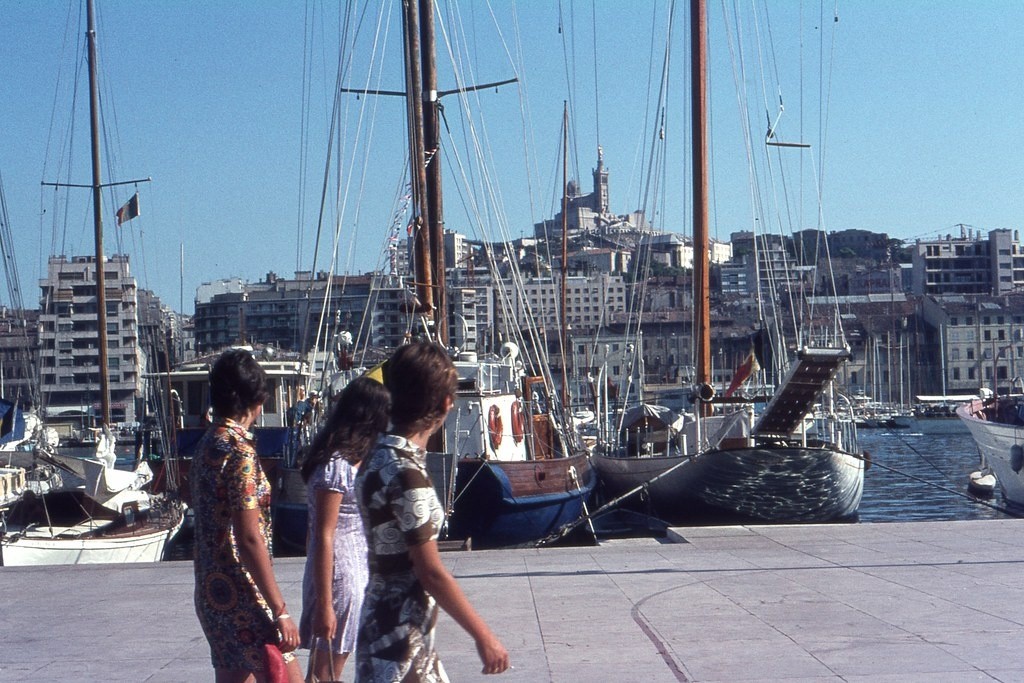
[289,638,293,641]
[315,632,320,635]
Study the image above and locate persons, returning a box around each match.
[299,341,509,683]
[194,348,305,683]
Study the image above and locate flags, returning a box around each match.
[725,351,760,400]
[115,193,140,226]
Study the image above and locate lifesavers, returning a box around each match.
[511,401,526,443]
[698,383,715,403]
[863,449,871,472]
[488,404,503,449]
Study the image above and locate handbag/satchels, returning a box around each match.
[304,632,350,683]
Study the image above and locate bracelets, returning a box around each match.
[271,603,290,623]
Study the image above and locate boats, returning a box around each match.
[956,336,1023,509]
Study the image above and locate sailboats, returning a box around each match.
[2,4,917,565]
